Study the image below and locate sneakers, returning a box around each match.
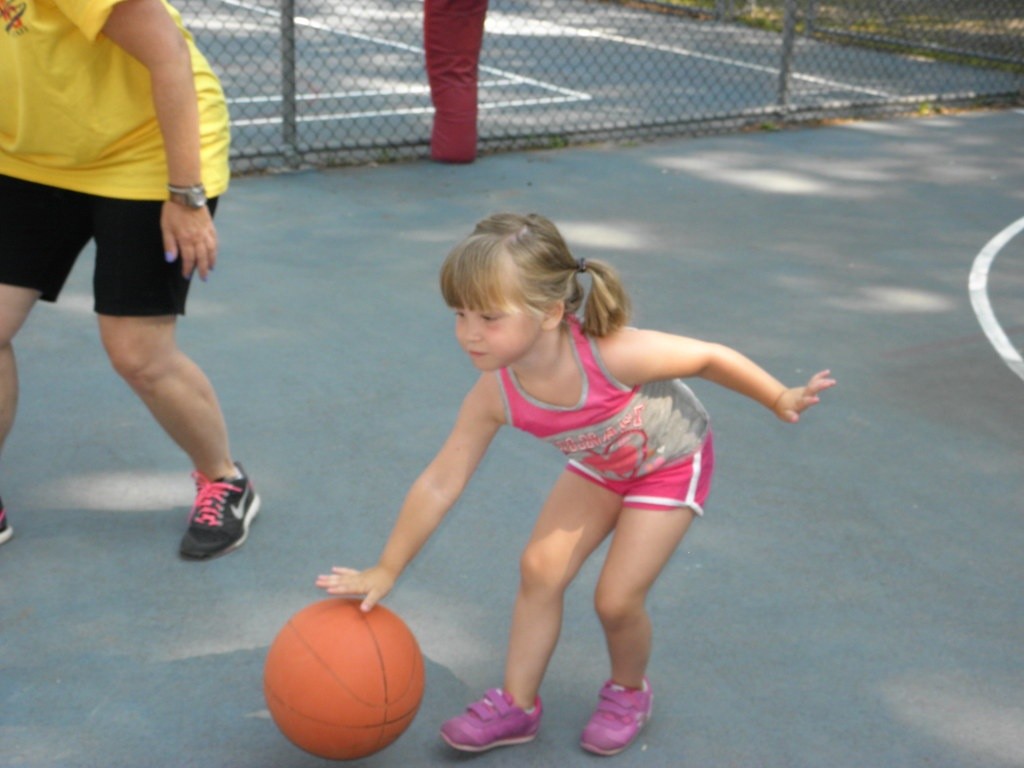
[441,688,542,751]
[581,675,653,755]
[178,461,260,560]
[0,498,13,544]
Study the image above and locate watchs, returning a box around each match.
[168,181,206,209]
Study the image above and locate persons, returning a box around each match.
[0,1,263,561]
[316,212,835,759]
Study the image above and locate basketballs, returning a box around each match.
[263,599,424,761]
